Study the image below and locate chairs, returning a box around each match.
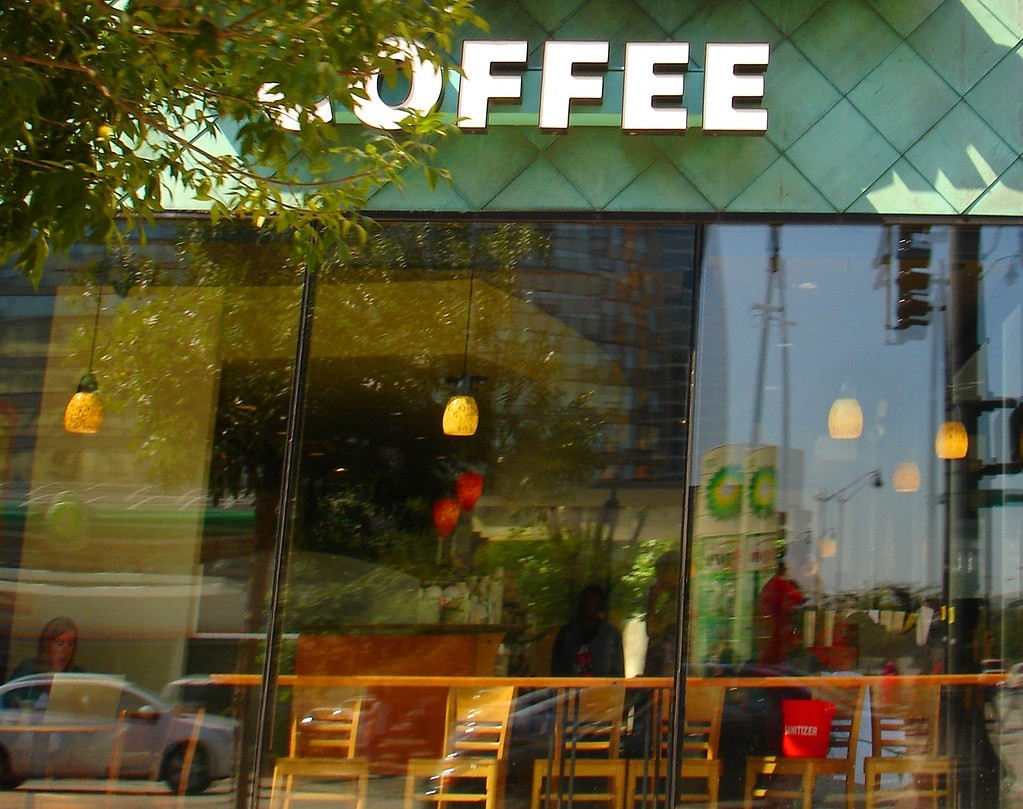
[531,685,627,809]
[404,684,519,809]
[744,684,870,809]
[863,683,961,809]
[270,685,367,808]
[625,685,727,808]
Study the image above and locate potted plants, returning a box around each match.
[620,540,672,679]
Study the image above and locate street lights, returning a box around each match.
[813,469,885,605]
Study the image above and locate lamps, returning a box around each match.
[441,226,479,435]
[798,317,968,579]
[66,269,101,434]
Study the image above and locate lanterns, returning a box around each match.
[65,392,104,434]
[433,498,460,540]
[456,472,483,512]
[828,397,864,439]
[893,460,921,493]
[442,396,479,436]
[935,421,968,459]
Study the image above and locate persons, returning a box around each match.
[757,541,806,678]
[0,616,84,709]
[549,583,625,678]
[643,551,682,677]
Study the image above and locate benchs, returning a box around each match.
[209,675,1009,809]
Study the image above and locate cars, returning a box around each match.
[0,671,245,796]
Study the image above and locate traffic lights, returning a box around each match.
[897,222,929,333]
